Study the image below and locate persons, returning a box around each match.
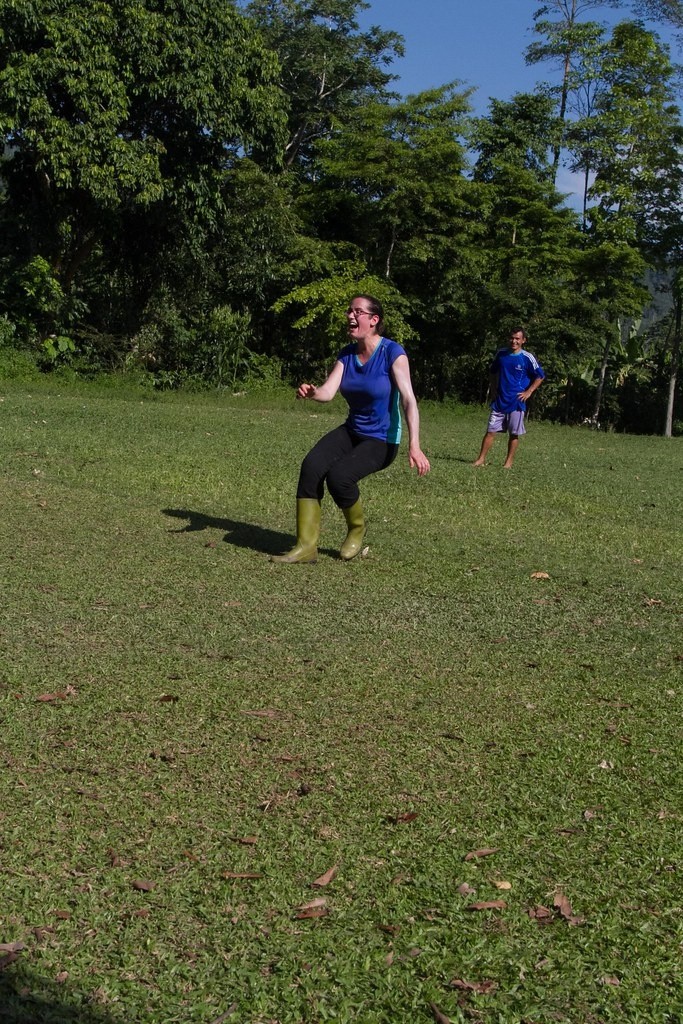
[472,327,545,469]
[269,293,430,565]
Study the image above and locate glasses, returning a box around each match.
[347,307,371,316]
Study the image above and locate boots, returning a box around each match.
[340,496,369,561]
[270,498,321,564]
